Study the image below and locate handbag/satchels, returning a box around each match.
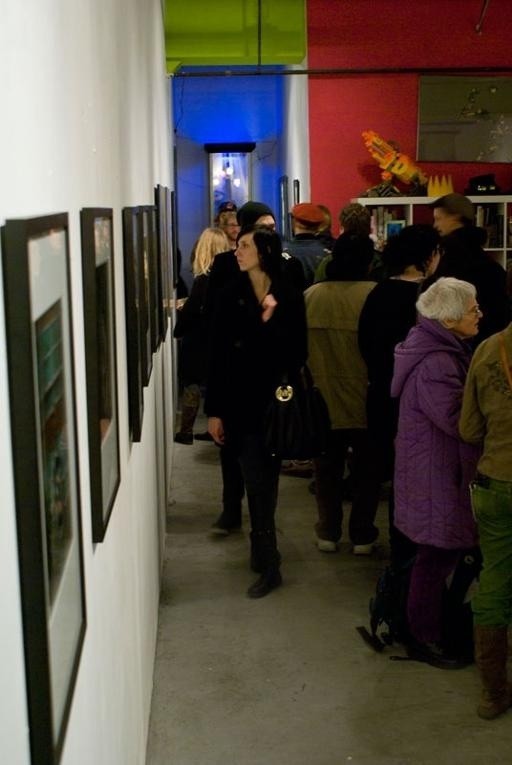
[258,364,332,464]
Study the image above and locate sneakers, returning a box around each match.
[311,529,337,552]
[194,430,215,442]
[174,432,193,445]
[247,569,282,598]
[353,541,375,555]
[207,511,242,535]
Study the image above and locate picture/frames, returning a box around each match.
[121,205,168,443]
[80,207,121,543]
[1,211,88,764]
[276,175,289,240]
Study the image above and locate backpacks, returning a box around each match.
[355,566,475,669]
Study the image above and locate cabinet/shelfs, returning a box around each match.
[349,193,512,271]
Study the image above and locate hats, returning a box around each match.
[292,203,326,227]
[236,200,275,231]
[214,201,237,222]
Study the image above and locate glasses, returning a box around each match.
[464,307,479,317]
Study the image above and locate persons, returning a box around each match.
[177,201,333,599]
[305,192,511,720]
[213,161,243,223]
